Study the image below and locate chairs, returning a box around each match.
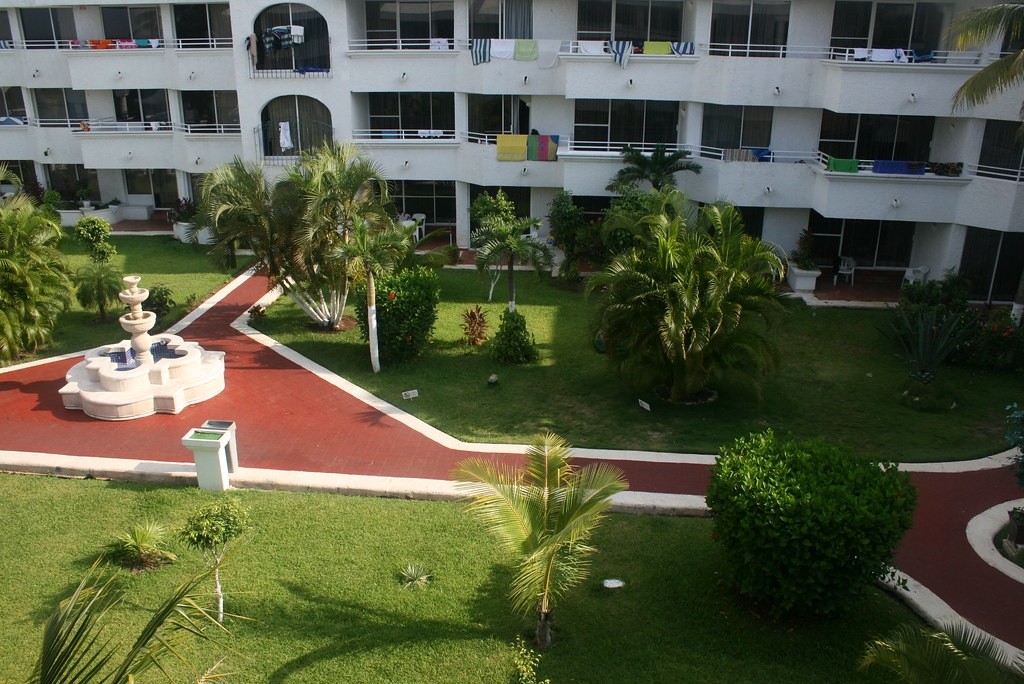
[833,256,856,288]
[520,231,538,242]
[401,220,419,244]
[901,264,931,287]
[546,229,559,251]
[410,213,426,240]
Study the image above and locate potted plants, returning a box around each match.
[1008,507,1024,545]
[76,187,94,208]
[786,227,822,292]
